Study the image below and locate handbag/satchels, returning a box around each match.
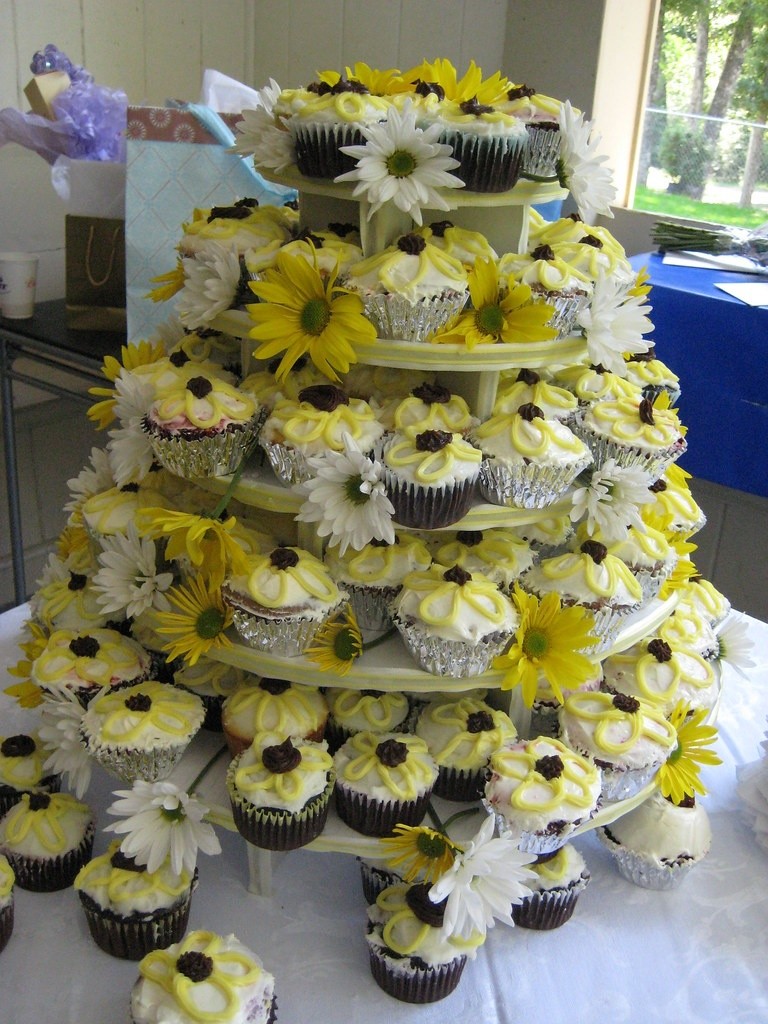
[63,211,128,334]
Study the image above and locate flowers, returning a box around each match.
[4,58,757,940]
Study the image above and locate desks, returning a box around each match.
[620,251,768,503]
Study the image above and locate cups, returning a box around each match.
[0,253,40,320]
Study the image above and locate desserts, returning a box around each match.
[0,55,732,1024]
